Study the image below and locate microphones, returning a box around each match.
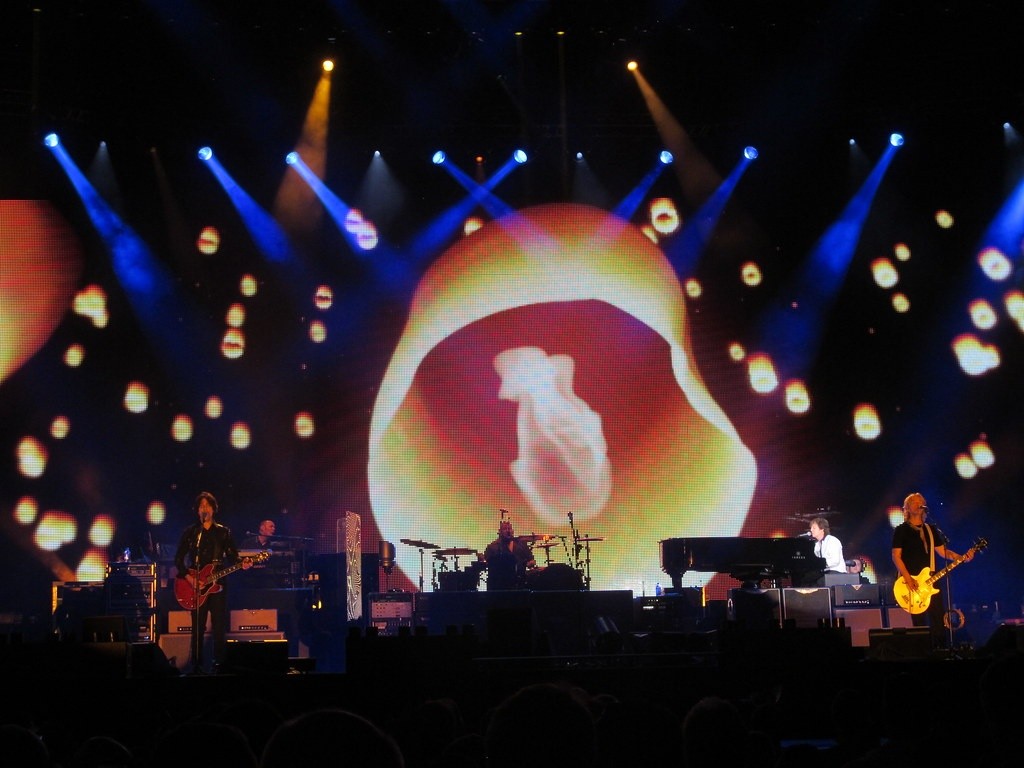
[798,531,812,536]
[918,504,929,509]
[203,512,207,521]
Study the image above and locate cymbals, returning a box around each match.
[432,547,478,555]
[400,538,441,550]
[535,542,560,548]
[576,537,607,542]
[517,535,556,541]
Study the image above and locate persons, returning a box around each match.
[595,631,626,666]
[849,556,870,584]
[0,696,484,768]
[485,665,825,768]
[127,654,167,673]
[477,522,536,590]
[809,518,846,587]
[237,520,279,609]
[974,647,991,657]
[803,651,1024,768]
[877,643,905,657]
[892,493,974,649]
[175,490,253,676]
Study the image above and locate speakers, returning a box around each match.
[159,610,294,670]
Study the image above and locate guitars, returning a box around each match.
[893,536,989,615]
[173,550,272,612]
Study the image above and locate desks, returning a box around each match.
[435,589,632,650]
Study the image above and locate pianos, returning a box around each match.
[657,535,827,591]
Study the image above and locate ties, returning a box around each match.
[908,521,928,554]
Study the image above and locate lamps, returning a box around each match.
[586,613,624,669]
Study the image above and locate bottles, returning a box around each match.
[386,619,411,633]
[208,659,223,674]
[817,618,846,631]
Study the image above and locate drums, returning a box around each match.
[526,563,586,591]
